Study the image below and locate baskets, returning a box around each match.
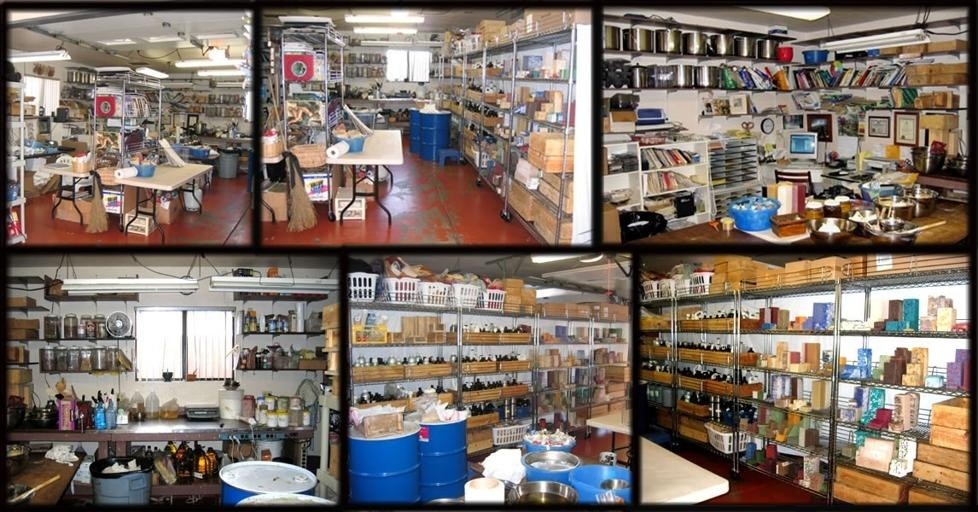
[417,281,452,308]
[449,283,482,310]
[71,158,91,173]
[347,272,380,304]
[703,422,752,455]
[382,277,421,305]
[470,144,499,168]
[259,140,283,158]
[96,167,122,186]
[288,144,328,169]
[642,271,715,303]
[489,424,529,446]
[352,324,389,344]
[473,288,507,314]
[453,34,483,57]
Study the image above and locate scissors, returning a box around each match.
[742,121,754,132]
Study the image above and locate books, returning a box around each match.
[725,65,911,90]
[642,148,694,170]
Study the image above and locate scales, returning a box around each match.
[186,405,219,421]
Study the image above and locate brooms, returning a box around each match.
[267,75,318,233]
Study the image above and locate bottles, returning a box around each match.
[145,441,231,480]
[465,81,505,118]
[242,306,297,333]
[38,313,125,371]
[451,321,531,423]
[345,52,387,77]
[350,353,457,405]
[255,395,312,428]
[94,388,161,429]
[641,308,760,423]
[205,93,245,118]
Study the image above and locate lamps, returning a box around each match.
[136,65,172,80]
[205,258,341,294]
[6,46,71,65]
[51,256,200,294]
[360,39,444,48]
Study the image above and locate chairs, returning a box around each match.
[772,168,816,196]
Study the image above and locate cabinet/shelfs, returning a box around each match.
[704,135,764,219]
[603,136,644,217]
[36,273,132,374]
[314,388,340,501]
[279,18,351,223]
[637,138,715,226]
[7,273,51,367]
[345,292,629,460]
[5,79,35,245]
[230,290,326,374]
[90,70,164,232]
[7,418,320,501]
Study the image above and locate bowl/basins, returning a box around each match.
[802,50,830,64]
[910,145,969,176]
[509,430,633,505]
[6,405,56,476]
[718,183,940,244]
[162,372,174,380]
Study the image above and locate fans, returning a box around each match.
[104,308,133,340]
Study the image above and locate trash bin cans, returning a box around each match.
[89,456,154,505]
[217,148,240,178]
[619,211,667,242]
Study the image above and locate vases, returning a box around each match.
[180,182,204,214]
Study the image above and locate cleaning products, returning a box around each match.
[104,393,116,429]
[93,401,104,430]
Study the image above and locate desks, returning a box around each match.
[906,166,968,195]
[70,157,92,174]
[259,156,288,227]
[320,127,406,225]
[622,193,969,245]
[6,447,89,502]
[113,160,213,245]
[580,402,630,468]
[370,96,430,108]
[43,154,96,228]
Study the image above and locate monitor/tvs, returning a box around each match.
[787,132,818,165]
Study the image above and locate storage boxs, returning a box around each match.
[7,290,39,411]
[101,187,127,215]
[601,201,623,243]
[498,275,538,306]
[333,185,367,223]
[329,438,341,481]
[431,10,575,246]
[262,137,284,158]
[148,196,181,225]
[49,190,91,223]
[124,204,157,237]
[9,89,35,116]
[344,173,377,201]
[263,182,292,225]
[876,41,968,113]
[319,298,341,399]
[301,167,335,201]
[641,253,971,506]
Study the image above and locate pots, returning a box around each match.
[603,24,794,62]
[628,62,721,88]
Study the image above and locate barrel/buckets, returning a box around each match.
[523,436,576,452]
[410,107,421,153]
[219,460,317,505]
[145,389,159,420]
[237,493,337,505]
[568,464,632,505]
[348,422,421,502]
[521,450,581,486]
[419,110,451,160]
[127,390,145,420]
[417,410,468,500]
